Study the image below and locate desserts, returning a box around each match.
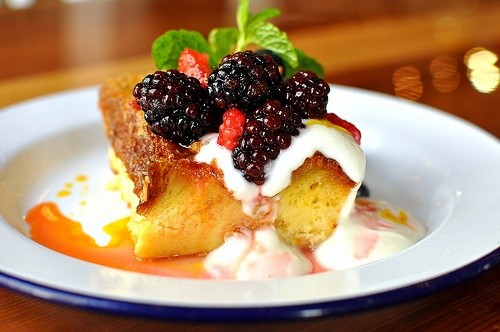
[97,0,428,280]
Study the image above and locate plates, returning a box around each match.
[2,86,500,324]
